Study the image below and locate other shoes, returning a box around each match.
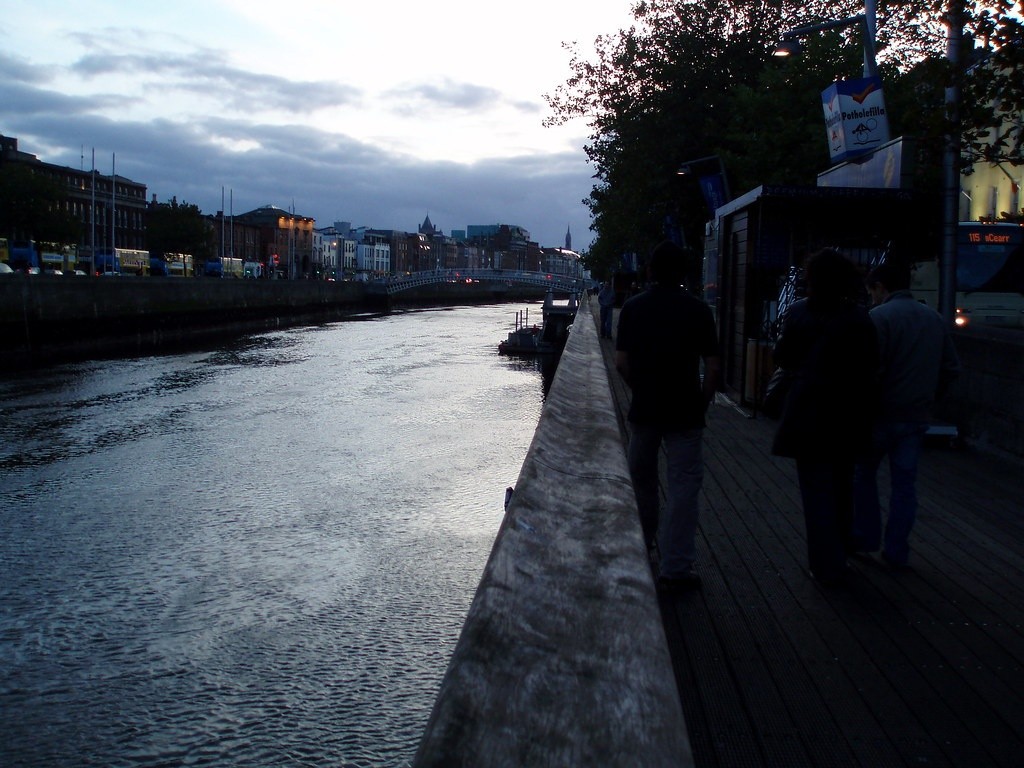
[601,335,605,339]
[657,572,703,594]
[607,336,612,339]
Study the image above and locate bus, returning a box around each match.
[911,220,1024,331]
[149,251,194,278]
[206,256,242,278]
[95,247,151,275]
[10,240,80,274]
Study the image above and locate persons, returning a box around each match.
[771,248,961,582]
[613,253,722,591]
[592,281,614,340]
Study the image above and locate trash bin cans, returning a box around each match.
[744,337,773,401]
